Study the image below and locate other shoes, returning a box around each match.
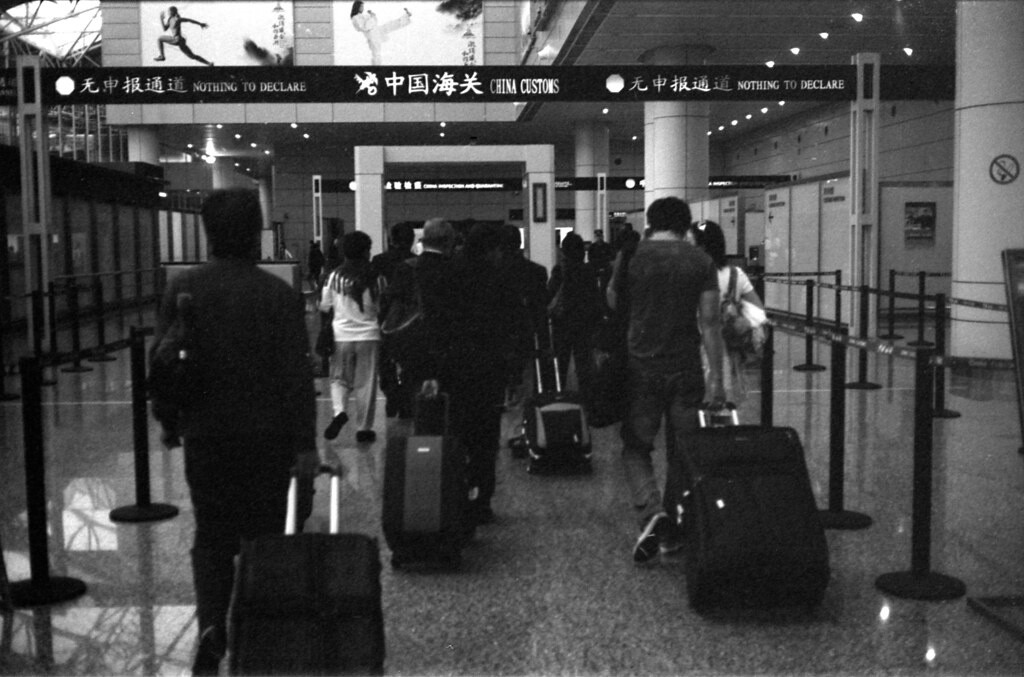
[476,508,497,521]
[356,430,376,442]
[193,616,227,677]
[325,412,348,439]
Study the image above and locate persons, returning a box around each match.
[315,231,381,445]
[149,186,322,677]
[350,0,412,65]
[72,240,83,303]
[684,220,772,427]
[153,6,214,66]
[547,231,598,407]
[495,225,549,407]
[588,222,640,279]
[306,241,323,291]
[450,224,532,522]
[606,197,726,563]
[378,218,473,542]
[370,222,420,270]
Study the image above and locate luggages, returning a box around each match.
[227,466,384,677]
[522,317,593,475]
[383,391,478,569]
[681,402,831,622]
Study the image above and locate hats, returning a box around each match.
[419,218,456,239]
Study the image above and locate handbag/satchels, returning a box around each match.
[149,272,193,435]
[721,265,752,351]
[315,306,335,356]
[380,257,430,371]
[546,261,571,325]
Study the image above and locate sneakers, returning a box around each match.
[659,536,685,553]
[632,509,671,566]
